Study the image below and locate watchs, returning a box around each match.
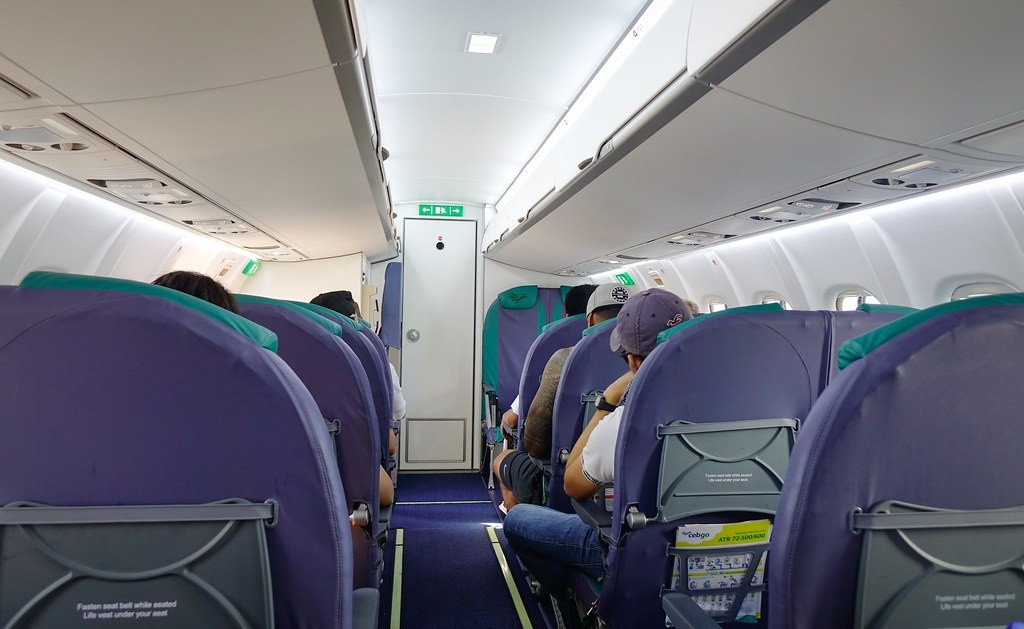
[594,393,617,413]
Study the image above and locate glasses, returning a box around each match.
[619,350,644,365]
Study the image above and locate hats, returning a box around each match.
[610,288,694,355]
[586,283,638,318]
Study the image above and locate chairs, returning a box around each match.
[1,271,1023,629]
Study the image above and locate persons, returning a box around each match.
[379,464,394,506]
[498,284,599,514]
[390,427,396,456]
[503,288,697,611]
[309,290,406,421]
[493,282,638,515]
